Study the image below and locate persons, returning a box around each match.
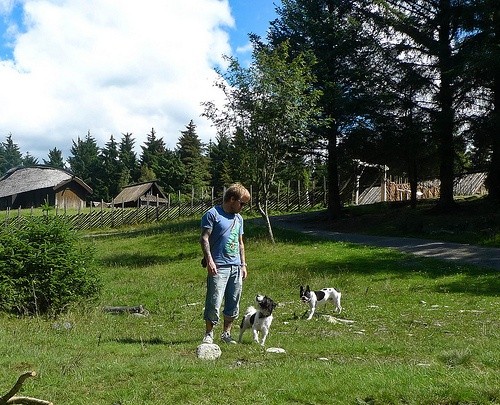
[201,185,250,344]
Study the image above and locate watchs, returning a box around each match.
[240,264,248,267]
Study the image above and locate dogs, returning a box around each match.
[300,285,342,321]
[239,294,277,347]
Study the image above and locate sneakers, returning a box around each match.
[221,333,236,345]
[202,332,213,344]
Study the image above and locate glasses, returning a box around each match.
[236,200,248,206]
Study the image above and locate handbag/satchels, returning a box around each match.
[200,256,207,268]
